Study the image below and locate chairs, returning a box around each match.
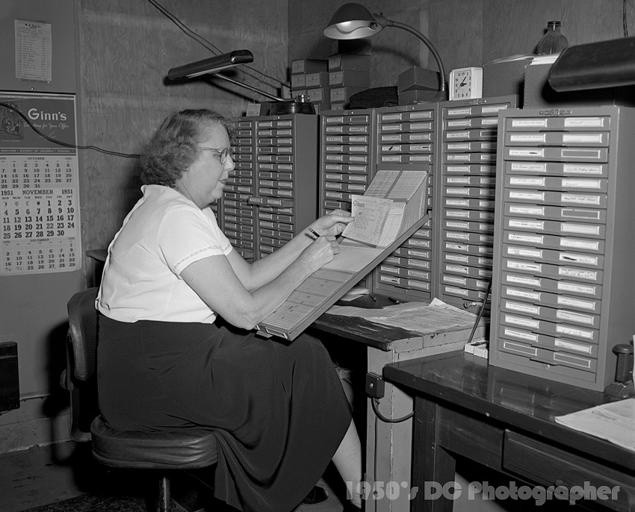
[65,285,218,510]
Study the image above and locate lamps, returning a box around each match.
[321,1,448,104]
[167,49,316,116]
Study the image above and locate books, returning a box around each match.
[554,397,635,455]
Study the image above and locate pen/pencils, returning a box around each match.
[388,297,400,304]
[369,294,376,302]
[308,227,320,237]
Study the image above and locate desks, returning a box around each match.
[382,346,634,511]
[83,248,505,511]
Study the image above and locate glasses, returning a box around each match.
[198,146,233,164]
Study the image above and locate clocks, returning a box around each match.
[448,67,483,102]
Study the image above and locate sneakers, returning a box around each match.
[342,499,364,512]
[303,484,329,504]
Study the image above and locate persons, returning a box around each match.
[93,109,363,512]
